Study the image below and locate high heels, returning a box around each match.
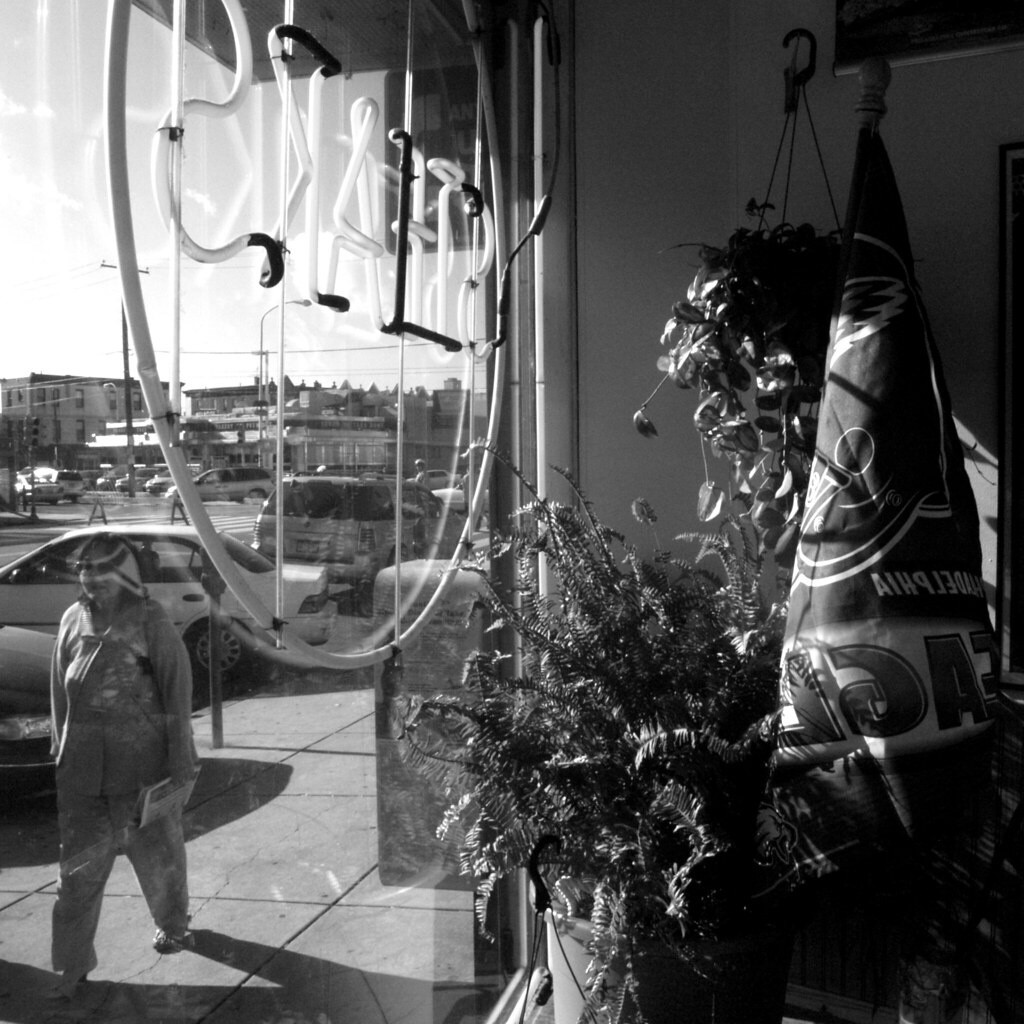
[43,951,98,999]
[153,914,191,953]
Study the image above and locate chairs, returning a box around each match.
[138,550,160,581]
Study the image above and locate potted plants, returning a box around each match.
[389,437,844,1024]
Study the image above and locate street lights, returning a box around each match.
[257,298,314,468]
[103,382,120,423]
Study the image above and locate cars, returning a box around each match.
[315,462,396,477]
[408,469,463,492]
[114,468,161,493]
[146,471,175,493]
[1,625,62,811]
[1,525,339,695]
[430,476,491,512]
[96,465,129,492]
[14,474,64,506]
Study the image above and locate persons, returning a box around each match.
[415,460,430,488]
[48,534,199,1008]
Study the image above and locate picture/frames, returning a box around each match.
[994,142,1024,694]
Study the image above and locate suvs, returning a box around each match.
[165,467,276,505]
[252,463,468,617]
[30,466,86,503]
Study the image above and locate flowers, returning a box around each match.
[630,199,847,525]
